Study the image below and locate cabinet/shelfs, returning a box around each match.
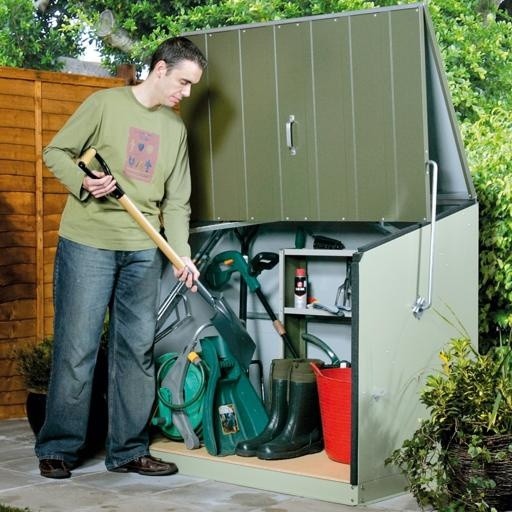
[280,249,355,319]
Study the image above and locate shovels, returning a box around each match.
[77,147,257,372]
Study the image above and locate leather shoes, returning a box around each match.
[109,456,178,475]
[39,459,71,478]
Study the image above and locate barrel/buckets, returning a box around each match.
[310,361,352,465]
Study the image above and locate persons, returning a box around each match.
[27,35,207,479]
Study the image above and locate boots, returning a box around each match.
[235,358,323,459]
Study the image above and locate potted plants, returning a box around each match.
[8,332,54,435]
[383,338,512,512]
